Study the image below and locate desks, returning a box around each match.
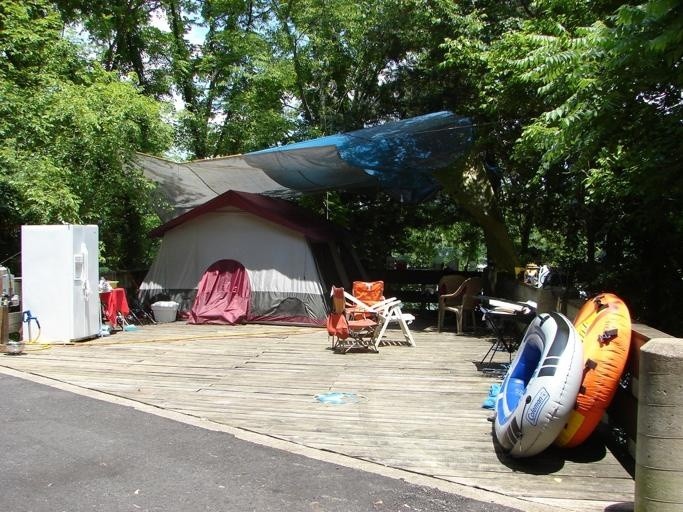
[478,306,518,368]
[100,288,126,330]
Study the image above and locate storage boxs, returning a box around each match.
[151,301,181,323]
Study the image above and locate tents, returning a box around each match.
[136,189,374,329]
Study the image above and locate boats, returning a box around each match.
[495,310,584,459]
[553,291,631,450]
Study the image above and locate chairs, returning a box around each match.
[437,275,482,332]
[328,281,415,355]
